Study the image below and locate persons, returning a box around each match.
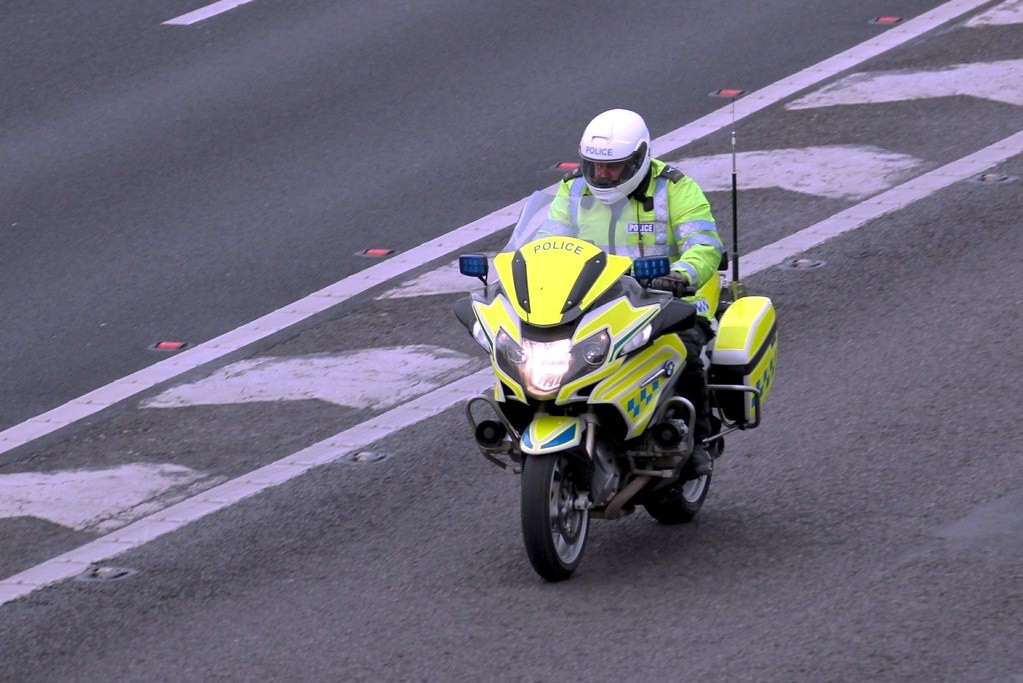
[530,108,726,461]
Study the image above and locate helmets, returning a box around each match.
[578,109,650,205]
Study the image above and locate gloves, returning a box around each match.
[652,271,689,299]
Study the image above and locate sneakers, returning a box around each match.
[683,446,711,480]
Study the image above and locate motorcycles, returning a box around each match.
[452,96,777,583]
